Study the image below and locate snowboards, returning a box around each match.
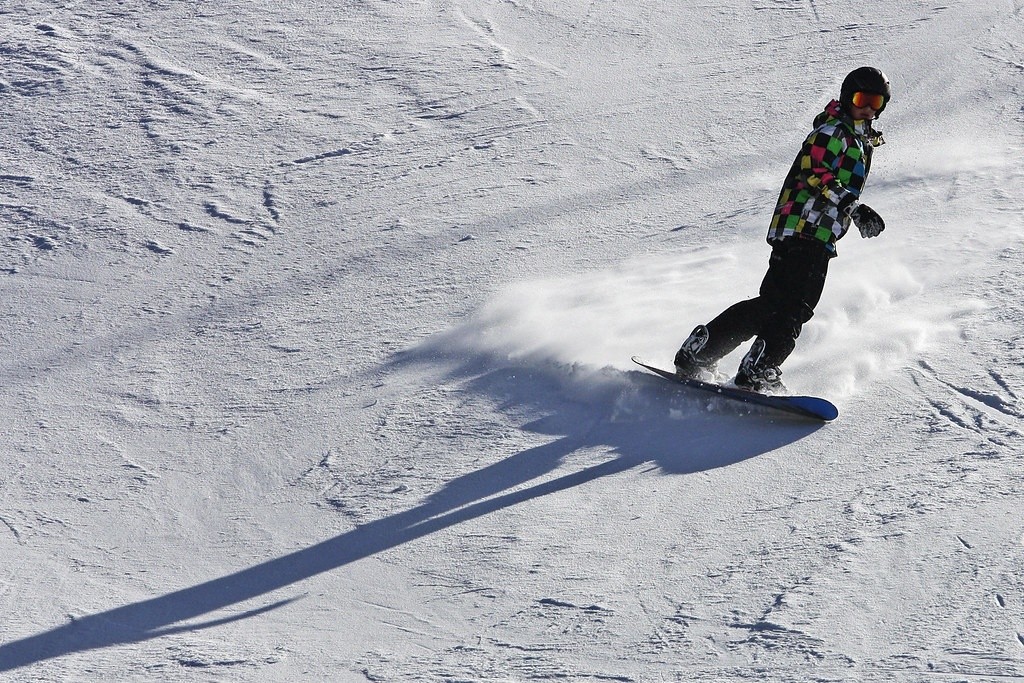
[629,349,842,423]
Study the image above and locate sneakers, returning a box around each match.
[734,336,790,395]
[673,324,731,385]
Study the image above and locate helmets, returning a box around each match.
[840,66,891,120]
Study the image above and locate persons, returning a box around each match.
[673,66,893,398]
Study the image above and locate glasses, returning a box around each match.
[847,91,885,112]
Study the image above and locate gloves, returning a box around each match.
[836,193,885,238]
